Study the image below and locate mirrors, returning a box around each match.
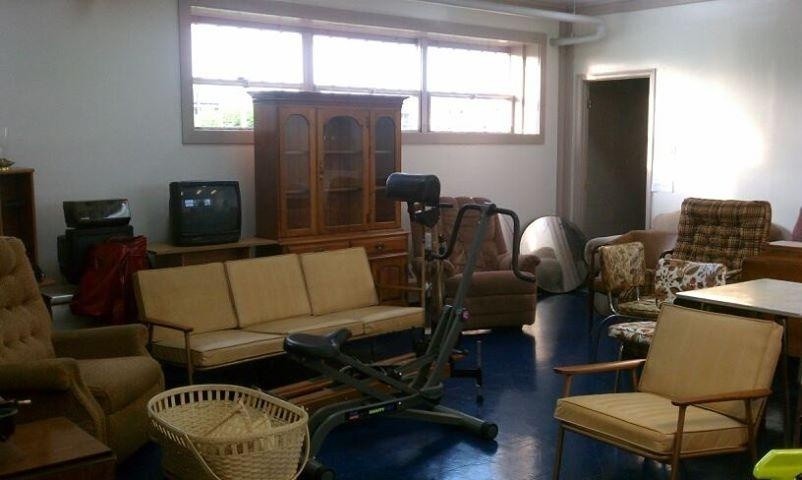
[521,214,591,294]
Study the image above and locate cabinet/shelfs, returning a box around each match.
[1,167,39,278]
[251,232,412,310]
[141,235,280,267]
[249,91,409,241]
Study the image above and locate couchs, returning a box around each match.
[131,245,430,391]
[422,196,540,337]
[582,198,794,323]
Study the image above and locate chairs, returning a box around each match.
[552,303,784,480]
[588,241,728,393]
[1,234,167,464]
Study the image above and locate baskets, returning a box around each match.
[146,383,310,479]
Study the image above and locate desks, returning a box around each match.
[740,241,802,282]
[674,278,802,357]
[2,408,119,479]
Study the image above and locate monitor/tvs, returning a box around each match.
[170,180,242,247]
